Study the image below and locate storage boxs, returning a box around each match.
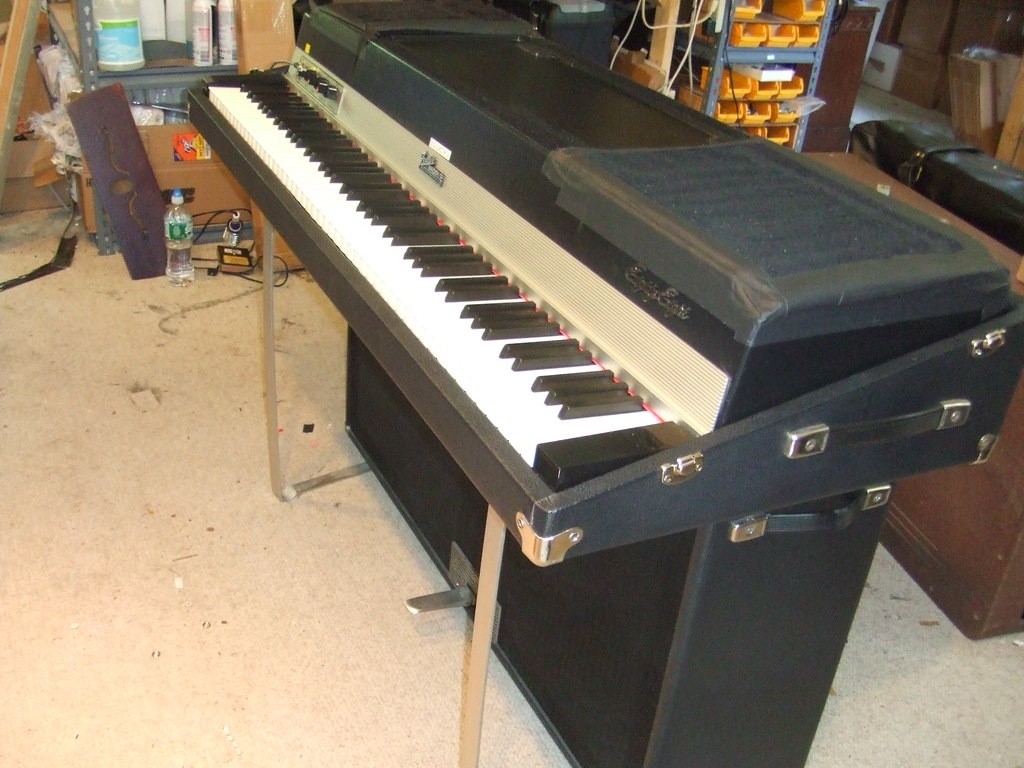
[0,0,304,273]
[863,0,1024,158]
[537,0,613,67]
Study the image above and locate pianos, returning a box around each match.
[181,1,1024,768]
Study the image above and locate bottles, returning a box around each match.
[163,188,195,287]
[91,0,148,72]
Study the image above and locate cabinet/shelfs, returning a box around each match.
[647,0,836,152]
